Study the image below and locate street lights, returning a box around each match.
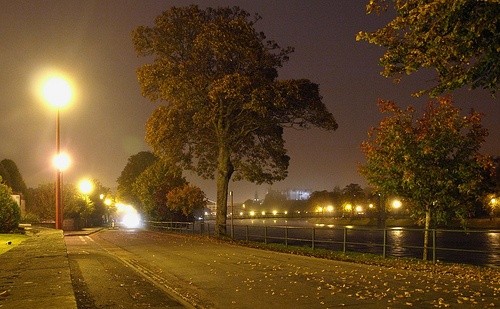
[44,76,71,229]
